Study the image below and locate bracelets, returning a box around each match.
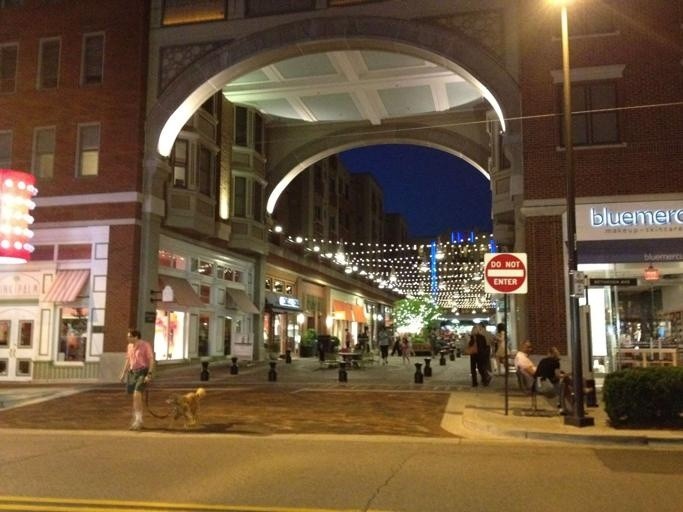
[147,372,152,375]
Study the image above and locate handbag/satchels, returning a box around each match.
[465,343,478,355]
[536,377,554,393]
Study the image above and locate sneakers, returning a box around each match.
[130,422,143,431]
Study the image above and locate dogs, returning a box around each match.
[165,387,207,432]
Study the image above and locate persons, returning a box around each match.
[120,330,153,431]
[346,325,411,366]
[424,321,576,416]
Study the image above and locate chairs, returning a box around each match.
[514,369,562,416]
